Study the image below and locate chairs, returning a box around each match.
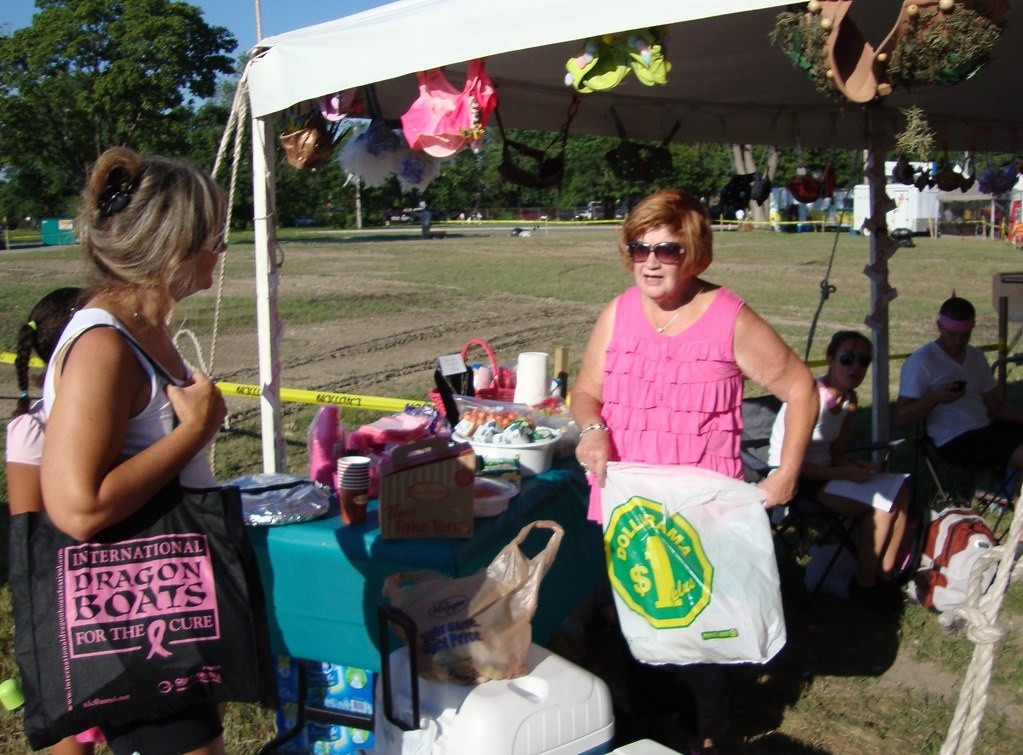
[739,395,906,611]
[907,405,1023,539]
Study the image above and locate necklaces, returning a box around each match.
[650,279,696,333]
[108,289,154,333]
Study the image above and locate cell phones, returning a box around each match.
[951,381,968,393]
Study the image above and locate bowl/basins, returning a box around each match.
[455,427,562,476]
[474,476,517,515]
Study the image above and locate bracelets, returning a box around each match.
[578,423,611,438]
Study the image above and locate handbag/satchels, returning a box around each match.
[378,436,475,538]
[380,517,564,687]
[6,323,280,751]
[583,458,787,667]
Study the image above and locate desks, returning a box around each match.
[247,451,609,751]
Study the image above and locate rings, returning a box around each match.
[581,461,586,467]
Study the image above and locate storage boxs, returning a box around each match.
[477,426,565,476]
[379,441,477,539]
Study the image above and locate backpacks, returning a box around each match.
[910,505,997,614]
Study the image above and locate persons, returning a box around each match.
[893,294,1023,512]
[943,204,1003,236]
[571,190,819,754]
[385,201,530,238]
[0,145,229,755]
[766,331,909,609]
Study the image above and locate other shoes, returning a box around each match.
[683,729,718,755]
[844,574,904,612]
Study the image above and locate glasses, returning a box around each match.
[627,239,686,264]
[202,238,228,256]
[834,351,872,368]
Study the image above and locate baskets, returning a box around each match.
[429,338,515,418]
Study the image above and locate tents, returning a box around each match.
[248,0,1023,475]
[933,180,1013,237]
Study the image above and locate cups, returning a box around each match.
[311,405,379,495]
[338,457,371,522]
